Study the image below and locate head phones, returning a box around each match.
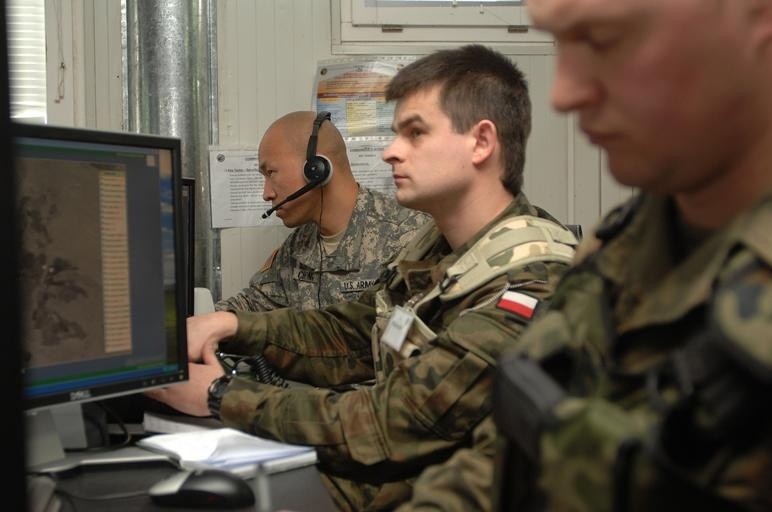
[302,111,333,189]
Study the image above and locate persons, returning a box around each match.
[145,41,582,488]
[411,0,772,512]
[215,111,433,313]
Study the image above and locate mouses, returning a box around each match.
[148,467,255,511]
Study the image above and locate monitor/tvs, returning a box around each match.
[8,121,189,480]
[181,177,197,317]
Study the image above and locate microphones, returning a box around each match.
[261,180,318,219]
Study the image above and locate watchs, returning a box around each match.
[207,375,233,420]
